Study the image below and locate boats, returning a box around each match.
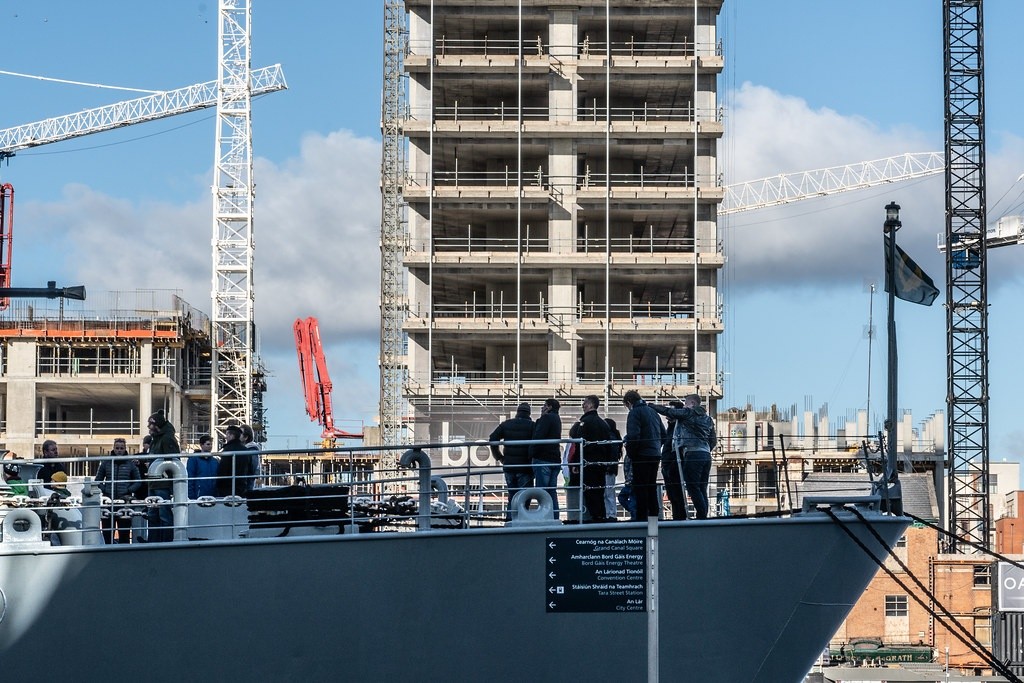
[1,430,915,683]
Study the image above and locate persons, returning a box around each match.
[490,402,536,519]
[240,425,260,490]
[604,418,623,521]
[661,401,687,520]
[95,438,141,543]
[3,463,28,497]
[187,436,219,500]
[572,394,611,518]
[624,391,668,521]
[648,394,717,518]
[529,398,561,520]
[34,440,71,545]
[878,641,884,649]
[840,645,845,659]
[614,455,637,519]
[214,425,252,498]
[133,409,181,542]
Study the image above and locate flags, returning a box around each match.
[883,234,940,306]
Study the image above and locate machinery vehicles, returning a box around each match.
[292,317,365,451]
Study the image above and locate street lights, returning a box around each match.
[883,200,902,483]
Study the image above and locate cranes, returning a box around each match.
[935,174,1024,254]
[0,63,290,161]
[718,150,945,215]
[941,0,994,587]
[206,0,257,454]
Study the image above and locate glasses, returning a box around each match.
[114,438,125,442]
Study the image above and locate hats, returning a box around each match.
[148,409,165,428]
[3,464,19,476]
[517,402,531,412]
[51,472,67,482]
[143,436,152,444]
